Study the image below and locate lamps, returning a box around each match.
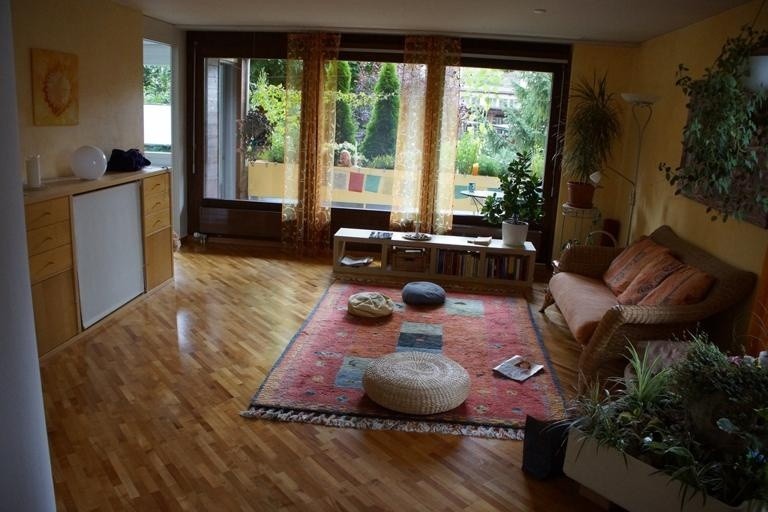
[589,91,661,246]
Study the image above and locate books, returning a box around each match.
[474,234,494,247]
[492,354,545,384]
[370,229,392,238]
[341,249,381,270]
[440,251,524,280]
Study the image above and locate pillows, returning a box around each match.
[603,234,717,308]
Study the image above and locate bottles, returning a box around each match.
[24,153,43,188]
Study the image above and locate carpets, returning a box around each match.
[237,278,570,441]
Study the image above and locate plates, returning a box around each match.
[402,234,433,240]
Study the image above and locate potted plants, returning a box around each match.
[481,151,546,247]
[239,105,273,168]
[551,70,626,209]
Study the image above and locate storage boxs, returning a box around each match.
[562,394,767,512]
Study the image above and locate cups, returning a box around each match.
[468,181,477,193]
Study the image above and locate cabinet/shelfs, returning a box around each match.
[23,171,173,359]
[333,227,537,298]
[559,203,602,251]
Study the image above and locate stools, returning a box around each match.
[362,351,471,416]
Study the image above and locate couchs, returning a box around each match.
[539,224,757,401]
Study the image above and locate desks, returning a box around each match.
[460,190,505,216]
